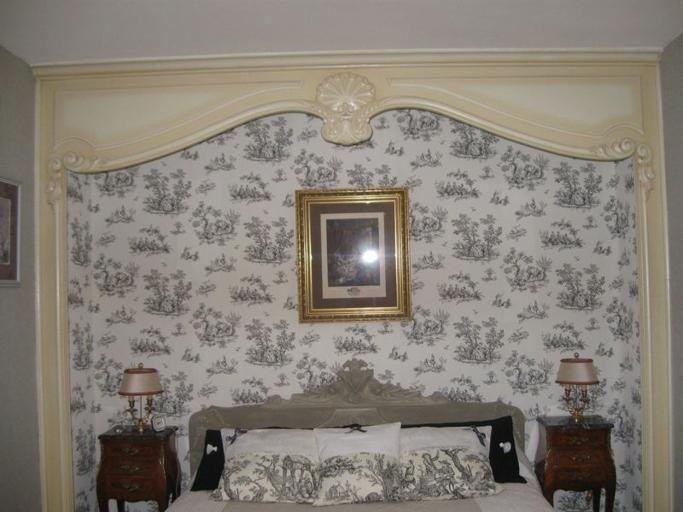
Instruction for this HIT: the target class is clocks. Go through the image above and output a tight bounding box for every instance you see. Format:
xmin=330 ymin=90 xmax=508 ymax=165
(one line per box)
xmin=151 ymin=414 xmax=167 ymax=432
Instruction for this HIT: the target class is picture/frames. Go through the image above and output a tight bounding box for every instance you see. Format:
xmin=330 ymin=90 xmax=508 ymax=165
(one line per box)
xmin=0 ymin=176 xmax=22 ymax=286
xmin=293 ymin=187 xmax=412 ymax=323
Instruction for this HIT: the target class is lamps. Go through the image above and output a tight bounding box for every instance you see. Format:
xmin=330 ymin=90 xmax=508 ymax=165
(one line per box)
xmin=118 ymin=362 xmax=164 ymax=433
xmin=555 ymin=352 xmax=601 ymax=424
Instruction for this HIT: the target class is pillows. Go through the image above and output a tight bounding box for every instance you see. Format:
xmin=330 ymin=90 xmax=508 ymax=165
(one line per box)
xmin=314 ymin=422 xmax=403 ymax=506
xmin=401 ymin=425 xmax=508 ymax=501
xmin=192 ymin=430 xmax=225 ymax=491
xmin=208 ymin=427 xmax=318 ymax=503
xmin=400 ymin=415 xmax=526 ymax=486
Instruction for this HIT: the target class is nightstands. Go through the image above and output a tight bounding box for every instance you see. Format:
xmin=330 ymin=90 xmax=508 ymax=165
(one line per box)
xmin=533 ymin=415 xmax=616 ymax=512
xmin=96 ymin=424 xmax=181 ymax=512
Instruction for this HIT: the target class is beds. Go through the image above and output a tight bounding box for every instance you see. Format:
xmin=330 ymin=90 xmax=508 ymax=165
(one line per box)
xmin=160 ymin=358 xmax=559 ymax=512
